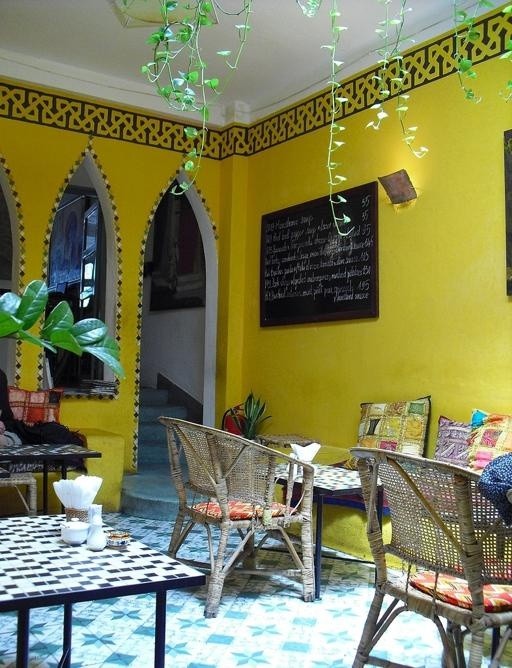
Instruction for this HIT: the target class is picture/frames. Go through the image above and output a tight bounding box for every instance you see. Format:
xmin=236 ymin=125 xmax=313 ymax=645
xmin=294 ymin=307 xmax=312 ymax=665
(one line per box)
xmin=46 ymin=195 xmax=89 ymax=292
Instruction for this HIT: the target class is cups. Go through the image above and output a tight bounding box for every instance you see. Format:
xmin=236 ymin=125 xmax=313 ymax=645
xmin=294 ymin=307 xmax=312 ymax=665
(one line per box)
xmin=60 ymin=507 xmax=92 ymax=546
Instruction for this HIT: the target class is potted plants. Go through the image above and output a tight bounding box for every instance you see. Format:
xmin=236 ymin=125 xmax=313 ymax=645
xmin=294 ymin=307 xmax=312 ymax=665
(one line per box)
xmin=227 ymin=387 xmax=271 ymax=442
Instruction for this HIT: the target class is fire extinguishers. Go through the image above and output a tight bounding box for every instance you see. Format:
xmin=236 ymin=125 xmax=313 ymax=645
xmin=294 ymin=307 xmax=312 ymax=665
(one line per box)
xmin=222 ymin=403 xmax=246 ymax=436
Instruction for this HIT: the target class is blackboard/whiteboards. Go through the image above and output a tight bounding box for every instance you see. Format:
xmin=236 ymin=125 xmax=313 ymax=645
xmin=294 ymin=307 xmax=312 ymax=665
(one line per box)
xmin=259 ymin=180 xmax=379 ymax=327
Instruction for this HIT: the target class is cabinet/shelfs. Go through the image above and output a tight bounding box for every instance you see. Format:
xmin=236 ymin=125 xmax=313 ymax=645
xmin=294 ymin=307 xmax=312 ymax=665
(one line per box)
xmin=80 ymin=200 xmax=108 ymax=379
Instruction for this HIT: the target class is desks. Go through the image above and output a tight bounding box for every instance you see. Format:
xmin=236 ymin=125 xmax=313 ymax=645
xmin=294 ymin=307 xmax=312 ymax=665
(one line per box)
xmin=257 ymin=462 xmax=383 ymax=601
xmin=0 ymin=442 xmax=102 ymax=513
xmin=0 ymin=515 xmax=206 ymax=668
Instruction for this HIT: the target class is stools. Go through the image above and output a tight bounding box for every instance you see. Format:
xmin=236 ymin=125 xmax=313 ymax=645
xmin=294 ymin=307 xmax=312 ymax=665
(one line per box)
xmin=0 ymin=471 xmax=36 ymax=518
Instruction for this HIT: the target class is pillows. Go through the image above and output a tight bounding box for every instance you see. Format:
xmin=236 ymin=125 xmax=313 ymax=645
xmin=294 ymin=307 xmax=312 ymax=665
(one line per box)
xmin=435 ymin=410 xmax=511 ymax=471
xmin=7 ymin=386 xmax=64 ymax=426
xmin=339 ymin=395 xmax=431 ymax=470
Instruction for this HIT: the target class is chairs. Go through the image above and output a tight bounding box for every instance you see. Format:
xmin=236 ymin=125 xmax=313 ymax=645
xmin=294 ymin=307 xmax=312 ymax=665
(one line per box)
xmin=156 ymin=414 xmax=314 ymax=618
xmin=348 ymin=445 xmax=512 ymax=668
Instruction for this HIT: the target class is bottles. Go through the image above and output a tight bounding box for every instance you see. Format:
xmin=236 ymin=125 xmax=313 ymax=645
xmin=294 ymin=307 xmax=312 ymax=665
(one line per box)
xmin=87 ymin=504 xmax=107 ymax=551
